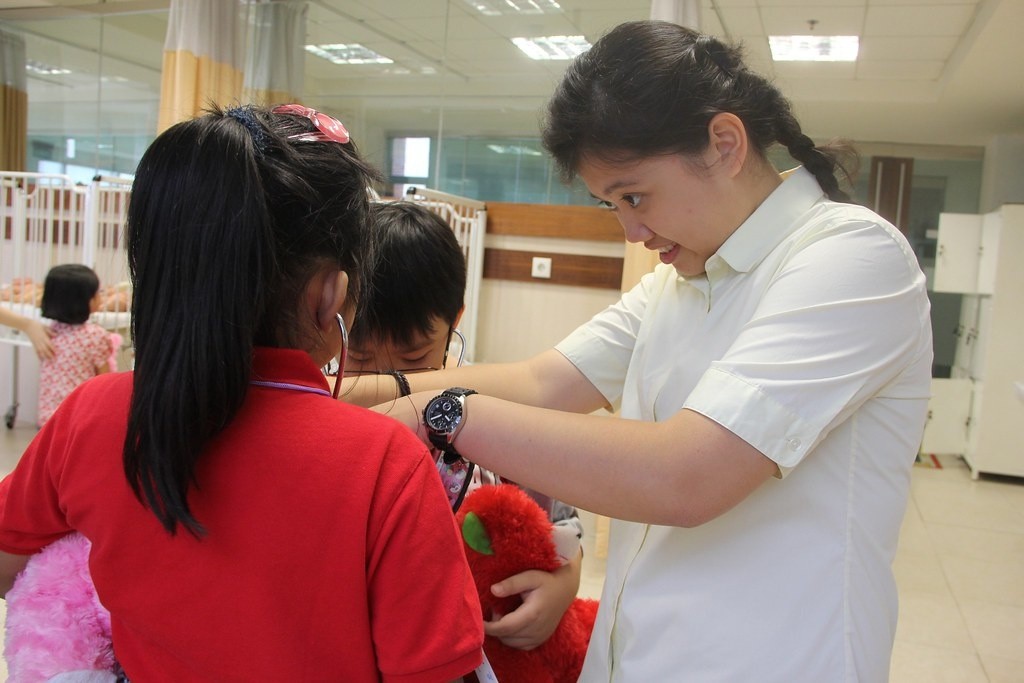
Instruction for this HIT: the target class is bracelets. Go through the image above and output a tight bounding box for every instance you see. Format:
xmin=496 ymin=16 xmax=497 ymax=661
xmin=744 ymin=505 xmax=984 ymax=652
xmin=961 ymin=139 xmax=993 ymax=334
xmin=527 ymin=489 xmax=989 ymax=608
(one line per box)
xmin=389 ymin=371 xmax=411 ymax=396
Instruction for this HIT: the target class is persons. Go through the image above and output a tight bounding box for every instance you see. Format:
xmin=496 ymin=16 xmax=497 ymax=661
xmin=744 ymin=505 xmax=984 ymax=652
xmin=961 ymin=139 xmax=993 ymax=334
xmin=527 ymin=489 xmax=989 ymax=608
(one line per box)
xmin=323 ymin=22 xmax=935 ymax=683
xmin=0 ymin=103 xmax=487 ymax=683
xmin=0 ymin=306 xmax=56 ymax=360
xmin=335 ymin=201 xmax=582 ymax=682
xmin=38 ymin=264 xmax=113 ymax=428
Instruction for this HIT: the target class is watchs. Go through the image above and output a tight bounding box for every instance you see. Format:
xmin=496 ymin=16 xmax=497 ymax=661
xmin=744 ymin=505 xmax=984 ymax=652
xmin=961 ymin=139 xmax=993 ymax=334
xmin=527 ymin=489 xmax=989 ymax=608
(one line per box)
xmin=422 ymin=387 xmax=477 ymax=464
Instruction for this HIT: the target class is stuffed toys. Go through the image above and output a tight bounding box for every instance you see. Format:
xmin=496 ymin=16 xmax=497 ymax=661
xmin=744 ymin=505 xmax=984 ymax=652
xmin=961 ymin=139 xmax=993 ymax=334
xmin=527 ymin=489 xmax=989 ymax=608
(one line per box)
xmin=4 ymin=532 xmax=127 ymax=683
xmin=108 ymin=334 xmax=122 ymax=372
xmin=457 ymin=484 xmax=600 ymax=683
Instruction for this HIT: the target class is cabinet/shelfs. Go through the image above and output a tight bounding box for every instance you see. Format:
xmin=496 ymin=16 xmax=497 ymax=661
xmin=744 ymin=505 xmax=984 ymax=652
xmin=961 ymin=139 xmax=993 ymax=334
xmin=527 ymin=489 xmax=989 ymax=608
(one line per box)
xmin=921 ymin=203 xmax=1024 ymax=482
